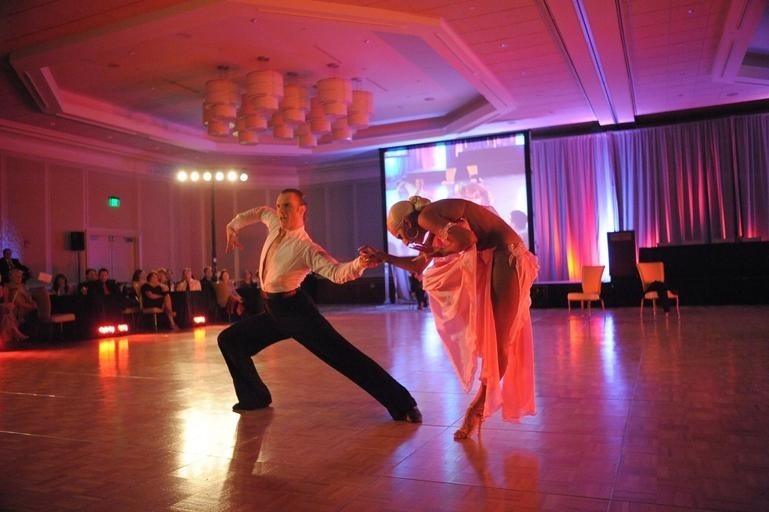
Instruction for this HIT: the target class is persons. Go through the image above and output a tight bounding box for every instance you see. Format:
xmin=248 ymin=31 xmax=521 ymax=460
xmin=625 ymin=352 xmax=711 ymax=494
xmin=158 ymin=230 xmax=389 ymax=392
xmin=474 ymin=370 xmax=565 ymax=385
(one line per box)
xmin=407 ymin=273 xmax=431 ymax=310
xmin=0 ymin=248 xmax=259 ymax=351
xmin=359 ymin=196 xmax=540 ymax=442
xmin=217 ymin=188 xmax=422 ymax=423
xmin=389 ymin=177 xmax=529 ymax=300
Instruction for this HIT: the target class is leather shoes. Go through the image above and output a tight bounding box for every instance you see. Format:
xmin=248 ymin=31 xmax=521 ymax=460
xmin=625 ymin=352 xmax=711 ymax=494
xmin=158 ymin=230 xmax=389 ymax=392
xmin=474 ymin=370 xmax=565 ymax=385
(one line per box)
xmin=404 ymin=405 xmax=422 ymax=423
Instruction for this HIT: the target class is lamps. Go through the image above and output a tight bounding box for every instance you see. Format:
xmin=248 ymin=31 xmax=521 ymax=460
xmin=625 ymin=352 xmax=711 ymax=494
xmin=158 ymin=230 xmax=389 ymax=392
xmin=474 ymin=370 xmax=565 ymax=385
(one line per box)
xmin=173 ymin=166 xmax=250 ymax=186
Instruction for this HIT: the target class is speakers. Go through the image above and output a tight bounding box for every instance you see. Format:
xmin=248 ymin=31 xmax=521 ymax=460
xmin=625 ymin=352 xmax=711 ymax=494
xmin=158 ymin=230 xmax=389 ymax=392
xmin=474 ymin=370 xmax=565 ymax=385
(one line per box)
xmin=71 ymin=232 xmax=84 ymax=251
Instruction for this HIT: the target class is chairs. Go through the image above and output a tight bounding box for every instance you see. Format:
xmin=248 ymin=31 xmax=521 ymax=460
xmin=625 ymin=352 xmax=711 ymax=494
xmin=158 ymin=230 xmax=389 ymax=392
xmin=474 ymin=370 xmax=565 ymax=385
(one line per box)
xmin=566 ymin=263 xmax=607 ymax=317
xmin=636 ymin=259 xmax=681 ymax=320
xmin=0 ymin=275 xmax=256 ymax=338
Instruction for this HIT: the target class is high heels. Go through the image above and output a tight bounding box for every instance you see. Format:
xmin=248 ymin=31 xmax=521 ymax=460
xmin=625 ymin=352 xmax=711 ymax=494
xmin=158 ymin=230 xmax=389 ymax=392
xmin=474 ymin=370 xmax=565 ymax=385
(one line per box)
xmin=453 ymin=403 xmax=484 ymax=440
